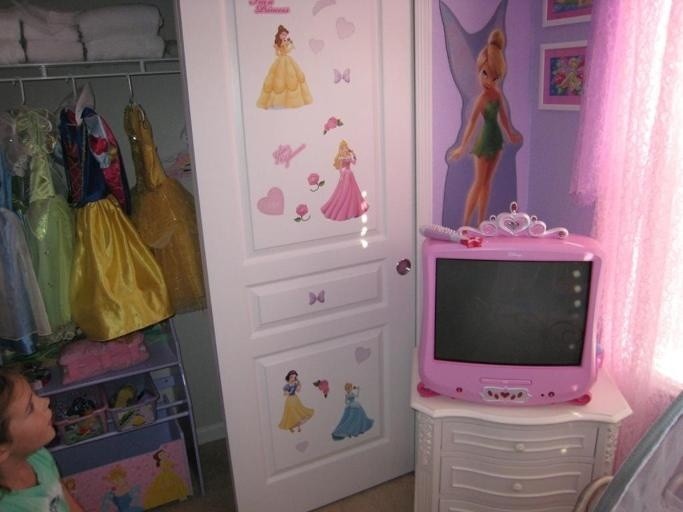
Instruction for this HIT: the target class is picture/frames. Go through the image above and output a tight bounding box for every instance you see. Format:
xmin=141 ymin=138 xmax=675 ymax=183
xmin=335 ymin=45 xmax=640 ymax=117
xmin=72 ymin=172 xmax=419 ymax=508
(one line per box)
xmin=541 ymin=0 xmax=593 ymax=27
xmin=539 ymin=39 xmax=591 ymax=111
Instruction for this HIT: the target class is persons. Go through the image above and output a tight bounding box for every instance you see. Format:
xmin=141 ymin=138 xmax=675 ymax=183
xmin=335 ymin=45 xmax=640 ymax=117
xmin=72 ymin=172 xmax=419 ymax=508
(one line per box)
xmin=146 ymin=450 xmax=189 ymax=509
xmin=331 ymin=383 xmax=374 ymax=440
xmin=276 ymin=370 xmax=315 ymax=432
xmin=447 ymin=27 xmax=521 ymax=226
xmin=0 ymin=361 xmax=86 ymax=512
xmin=559 ymin=57 xmax=579 ymax=96
xmin=320 ymin=141 xmax=371 ymax=222
xmin=61 ymin=477 xmax=82 ymax=511
xmin=257 ymin=23 xmax=312 ymax=111
xmin=101 ymin=468 xmax=145 ymax=509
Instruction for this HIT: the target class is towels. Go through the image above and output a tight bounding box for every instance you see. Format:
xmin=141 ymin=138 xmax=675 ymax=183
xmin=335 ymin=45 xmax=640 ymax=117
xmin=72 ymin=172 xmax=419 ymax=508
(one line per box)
xmin=0 ymin=4 xmax=166 ymax=65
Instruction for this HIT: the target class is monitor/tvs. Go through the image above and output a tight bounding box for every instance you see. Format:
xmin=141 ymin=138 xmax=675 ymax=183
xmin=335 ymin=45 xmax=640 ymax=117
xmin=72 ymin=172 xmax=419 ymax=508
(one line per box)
xmin=420 ymin=201 xmax=607 ymax=406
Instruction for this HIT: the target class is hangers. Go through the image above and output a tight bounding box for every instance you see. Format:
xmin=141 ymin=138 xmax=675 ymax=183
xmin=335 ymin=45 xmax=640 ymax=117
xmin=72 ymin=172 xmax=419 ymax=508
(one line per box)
xmin=10 ymin=76 xmax=145 ymax=133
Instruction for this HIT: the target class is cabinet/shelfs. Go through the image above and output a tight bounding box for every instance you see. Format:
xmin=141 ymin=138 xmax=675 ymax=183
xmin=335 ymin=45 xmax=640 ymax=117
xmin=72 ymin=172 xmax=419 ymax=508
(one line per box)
xmin=409 ymin=346 xmax=634 ymax=511
xmin=20 ymin=318 xmax=206 ymax=511
